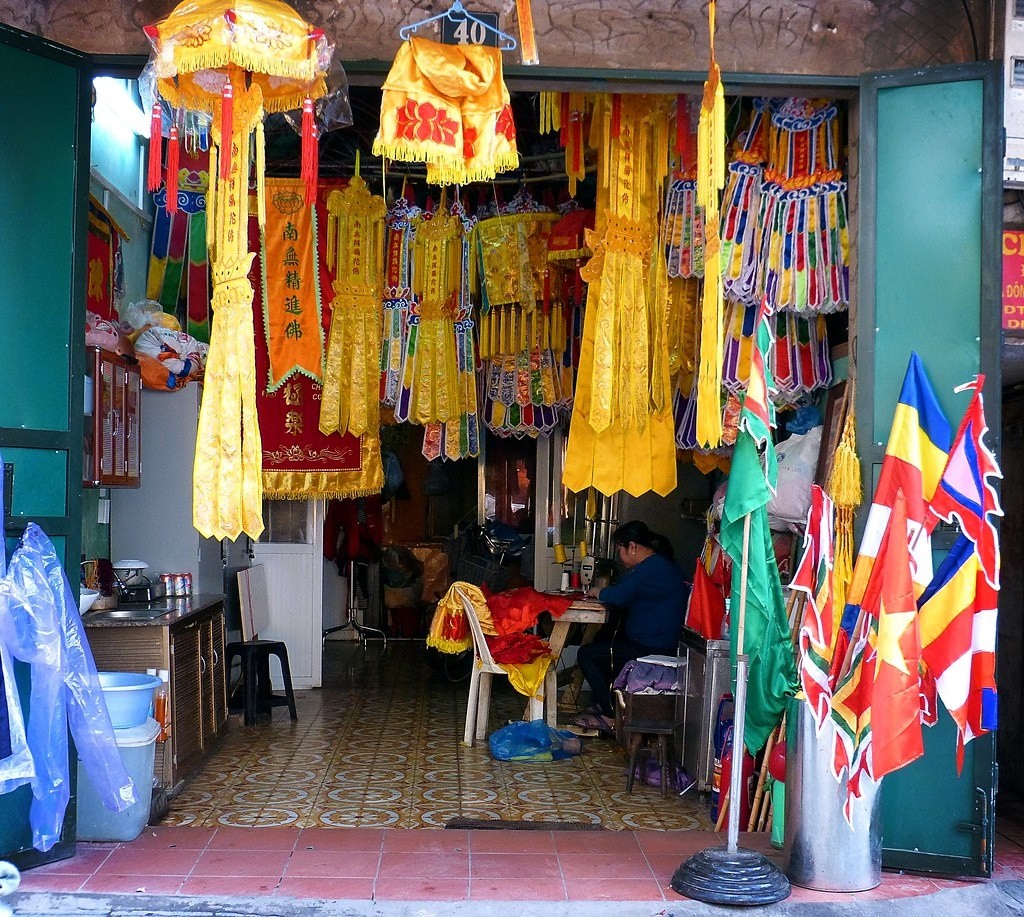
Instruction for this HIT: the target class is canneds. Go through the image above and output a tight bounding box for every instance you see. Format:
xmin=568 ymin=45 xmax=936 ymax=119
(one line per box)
xmin=160 ymin=573 xmax=193 ymax=596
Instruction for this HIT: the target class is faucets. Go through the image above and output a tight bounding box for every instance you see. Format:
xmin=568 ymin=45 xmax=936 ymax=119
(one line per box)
xmin=80 ymin=560 xmax=129 ymax=594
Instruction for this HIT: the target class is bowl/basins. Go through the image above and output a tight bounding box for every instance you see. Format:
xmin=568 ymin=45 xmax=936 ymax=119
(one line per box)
xmin=97 ymin=672 xmax=162 ymax=729
xmin=80 ymin=588 xmax=99 ymax=616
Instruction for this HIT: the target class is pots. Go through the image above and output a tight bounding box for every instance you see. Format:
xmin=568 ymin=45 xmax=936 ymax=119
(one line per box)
xmin=114 ymin=569 xmax=143 ymax=585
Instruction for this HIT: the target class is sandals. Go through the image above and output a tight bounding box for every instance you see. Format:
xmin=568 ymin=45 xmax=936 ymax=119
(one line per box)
xmin=575 ymin=714 xmax=618 ymax=736
xmin=580 ymin=702 xmax=604 ymax=714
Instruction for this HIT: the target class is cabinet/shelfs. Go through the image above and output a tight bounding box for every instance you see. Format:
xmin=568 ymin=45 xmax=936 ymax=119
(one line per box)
xmin=80 ymin=594 xmax=228 ymax=801
xmin=673 ymin=625 xmax=731 ymax=804
xmin=84 ymin=345 xmax=142 ymax=490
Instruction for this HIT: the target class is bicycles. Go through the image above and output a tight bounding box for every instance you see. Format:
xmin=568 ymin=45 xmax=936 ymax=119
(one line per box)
xmin=441 ymin=525 xmax=532 ymax=685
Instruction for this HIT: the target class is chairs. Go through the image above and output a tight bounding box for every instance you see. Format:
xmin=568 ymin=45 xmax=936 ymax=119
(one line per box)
xmin=453 ymin=585 xmax=544 ymax=746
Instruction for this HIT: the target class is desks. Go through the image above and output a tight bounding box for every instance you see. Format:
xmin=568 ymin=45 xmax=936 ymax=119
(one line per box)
xmin=505 ymin=588 xmax=609 ymax=739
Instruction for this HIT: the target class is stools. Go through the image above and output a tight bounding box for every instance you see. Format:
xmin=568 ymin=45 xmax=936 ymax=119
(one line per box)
xmin=622 ymin=721 xmax=684 ymax=799
xmin=225 ymin=640 xmax=298 ymax=728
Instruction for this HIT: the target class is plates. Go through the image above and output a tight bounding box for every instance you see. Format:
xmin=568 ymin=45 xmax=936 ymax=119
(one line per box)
xmin=113 ymin=559 xmax=148 ymax=568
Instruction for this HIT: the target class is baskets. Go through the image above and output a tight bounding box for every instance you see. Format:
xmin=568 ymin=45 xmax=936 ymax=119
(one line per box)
xmin=458 ymin=555 xmax=511 ymax=590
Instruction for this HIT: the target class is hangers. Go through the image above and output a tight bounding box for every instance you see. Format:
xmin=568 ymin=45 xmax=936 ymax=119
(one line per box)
xmin=399 ymin=0 xmax=517 ymax=51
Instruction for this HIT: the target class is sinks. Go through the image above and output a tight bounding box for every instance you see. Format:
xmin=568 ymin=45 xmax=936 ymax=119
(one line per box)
xmin=83 ymin=607 xmax=178 ymax=620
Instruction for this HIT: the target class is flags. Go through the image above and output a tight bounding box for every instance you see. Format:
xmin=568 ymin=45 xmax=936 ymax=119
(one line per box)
xmin=719 ymin=359 xmax=1005 ymax=827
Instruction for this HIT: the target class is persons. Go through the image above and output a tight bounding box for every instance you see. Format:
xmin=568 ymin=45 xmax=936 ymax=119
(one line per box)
xmin=572 ymin=520 xmax=690 ymax=731
